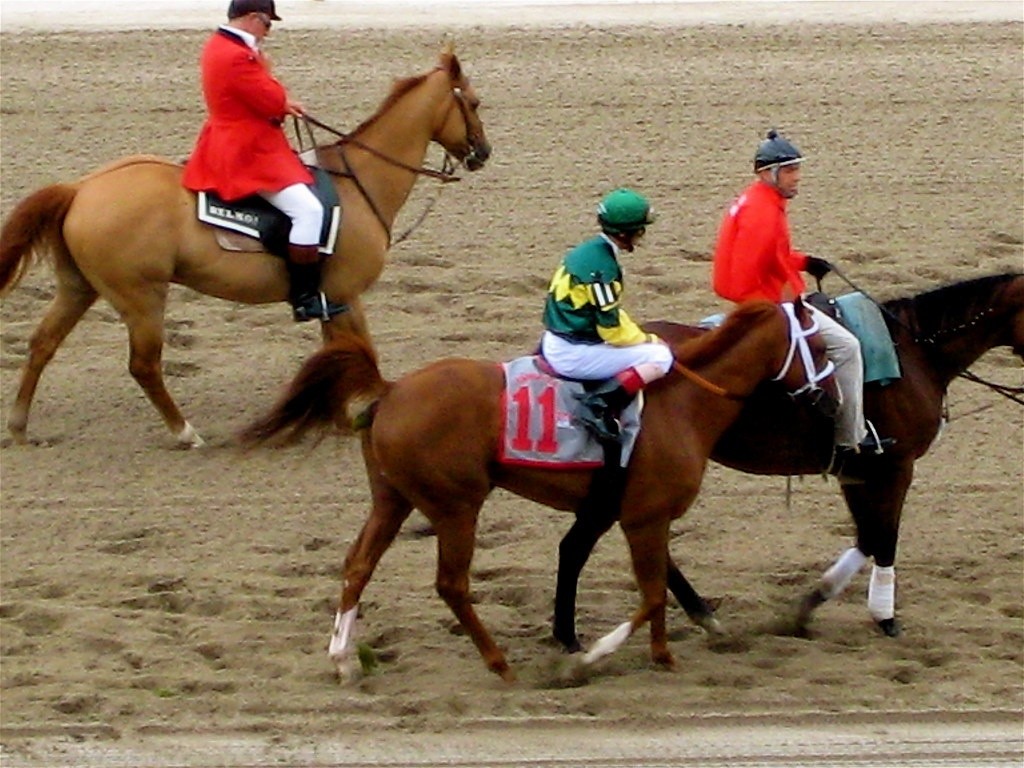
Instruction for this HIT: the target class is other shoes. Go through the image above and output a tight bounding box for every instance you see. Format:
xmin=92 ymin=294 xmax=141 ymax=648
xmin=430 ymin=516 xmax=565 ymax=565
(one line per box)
xmin=835 ymin=432 xmax=897 ymax=463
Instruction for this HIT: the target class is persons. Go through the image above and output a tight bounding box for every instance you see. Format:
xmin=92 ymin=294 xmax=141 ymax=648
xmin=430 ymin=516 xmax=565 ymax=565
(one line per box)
xmin=714 ymin=130 xmax=896 ymax=461
xmin=179 ymin=0 xmax=351 ymax=322
xmin=541 ymin=189 xmax=673 ymax=443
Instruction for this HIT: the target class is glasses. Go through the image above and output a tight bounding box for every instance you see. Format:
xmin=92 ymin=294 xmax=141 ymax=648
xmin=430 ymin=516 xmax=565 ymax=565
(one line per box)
xmin=633 ymin=227 xmax=646 ymax=236
xmin=258 ymin=14 xmax=273 ymax=31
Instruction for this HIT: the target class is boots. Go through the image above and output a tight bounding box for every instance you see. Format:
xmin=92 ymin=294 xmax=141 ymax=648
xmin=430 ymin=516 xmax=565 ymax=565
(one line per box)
xmin=287 ymin=243 xmax=350 ymax=322
xmin=572 ymin=367 xmax=648 ymax=444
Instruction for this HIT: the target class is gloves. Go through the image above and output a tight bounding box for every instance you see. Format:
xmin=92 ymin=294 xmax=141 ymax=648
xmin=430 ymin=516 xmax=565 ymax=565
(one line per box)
xmin=805 ymin=255 xmax=834 ymax=281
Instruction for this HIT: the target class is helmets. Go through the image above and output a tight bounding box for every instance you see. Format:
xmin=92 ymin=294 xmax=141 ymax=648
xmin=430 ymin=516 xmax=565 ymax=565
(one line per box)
xmin=597 ymin=187 xmax=660 ymax=234
xmin=228 ymin=0 xmax=282 ymax=21
xmin=753 ymin=128 xmax=806 ymax=173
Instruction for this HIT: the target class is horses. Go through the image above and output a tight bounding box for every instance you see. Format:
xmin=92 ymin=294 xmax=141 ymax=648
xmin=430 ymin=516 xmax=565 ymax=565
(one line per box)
xmin=1 ymin=45 xmax=492 ymax=450
xmin=197 ymin=294 xmax=843 ymax=687
xmin=407 ymin=273 xmax=1024 ymax=654
